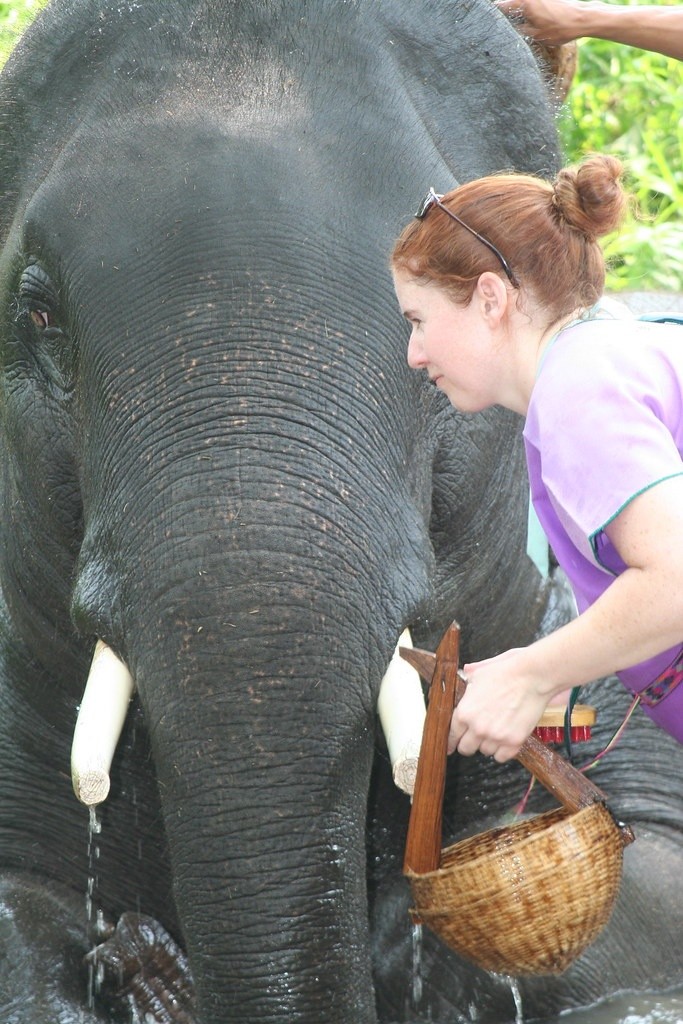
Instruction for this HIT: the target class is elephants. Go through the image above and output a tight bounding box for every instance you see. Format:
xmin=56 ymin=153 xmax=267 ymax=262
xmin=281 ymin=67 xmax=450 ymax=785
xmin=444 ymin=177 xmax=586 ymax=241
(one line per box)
xmin=0 ymin=0 xmax=682 ymax=1024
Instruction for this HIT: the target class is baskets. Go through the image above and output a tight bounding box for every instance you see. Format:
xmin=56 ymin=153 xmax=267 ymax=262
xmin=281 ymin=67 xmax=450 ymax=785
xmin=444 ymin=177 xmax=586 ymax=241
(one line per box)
xmin=397 ymin=623 xmax=633 ymax=978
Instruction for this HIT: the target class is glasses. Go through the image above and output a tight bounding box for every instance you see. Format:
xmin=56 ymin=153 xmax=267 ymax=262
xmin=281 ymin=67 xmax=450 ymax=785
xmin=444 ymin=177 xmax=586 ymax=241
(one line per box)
xmin=414 ymin=187 xmax=519 ymax=291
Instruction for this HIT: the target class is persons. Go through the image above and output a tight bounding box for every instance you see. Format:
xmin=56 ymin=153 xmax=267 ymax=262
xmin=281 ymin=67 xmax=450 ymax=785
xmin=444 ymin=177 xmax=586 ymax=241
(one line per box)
xmin=389 ymin=153 xmax=683 ymax=763
